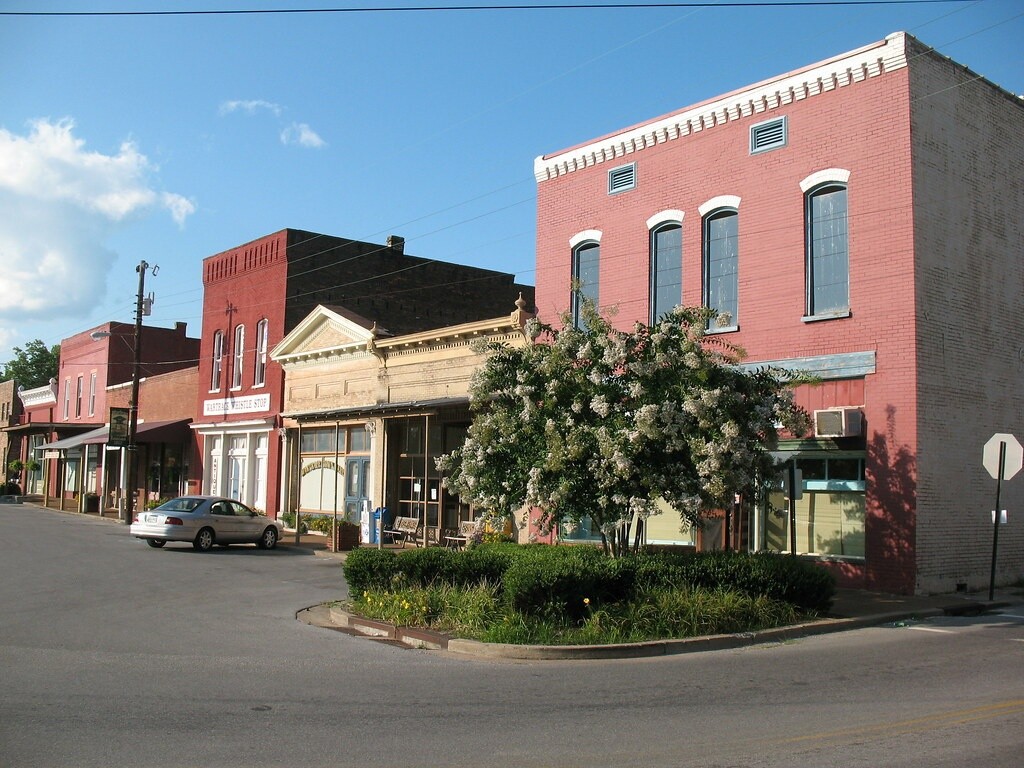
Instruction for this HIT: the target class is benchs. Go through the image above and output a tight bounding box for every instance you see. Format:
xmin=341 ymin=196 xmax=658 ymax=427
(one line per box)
xmin=382 ymin=516 xmax=420 ymax=548
xmin=443 ymin=521 xmax=476 ymax=551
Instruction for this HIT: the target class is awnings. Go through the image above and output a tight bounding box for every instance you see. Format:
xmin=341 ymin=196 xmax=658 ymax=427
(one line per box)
xmin=0 ymin=417 xmax=193 ymax=451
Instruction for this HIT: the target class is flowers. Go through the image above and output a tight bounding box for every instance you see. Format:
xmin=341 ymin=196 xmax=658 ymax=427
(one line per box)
xmin=75 ymin=491 xmax=100 ymax=504
xmin=328 ymin=520 xmax=355 ymax=539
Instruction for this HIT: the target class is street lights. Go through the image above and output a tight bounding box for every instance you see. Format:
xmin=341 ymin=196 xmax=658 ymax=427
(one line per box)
xmin=89 ymin=259 xmax=161 ymax=527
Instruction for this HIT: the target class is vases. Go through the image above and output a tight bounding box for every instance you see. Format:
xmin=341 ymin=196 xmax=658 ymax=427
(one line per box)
xmin=87 ymin=499 xmax=98 ymax=511
xmin=326 ymin=527 xmax=360 ymax=551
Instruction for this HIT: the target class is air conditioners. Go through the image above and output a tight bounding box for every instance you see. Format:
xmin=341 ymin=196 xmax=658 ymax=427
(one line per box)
xmin=814 ymin=409 xmax=862 ymax=437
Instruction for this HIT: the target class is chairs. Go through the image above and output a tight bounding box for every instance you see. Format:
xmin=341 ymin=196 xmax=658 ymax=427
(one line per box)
xmin=213 ymin=506 xmax=222 ymax=513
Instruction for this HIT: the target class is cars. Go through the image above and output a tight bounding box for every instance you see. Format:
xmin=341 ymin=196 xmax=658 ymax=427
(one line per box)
xmin=130 ymin=495 xmax=285 ymax=554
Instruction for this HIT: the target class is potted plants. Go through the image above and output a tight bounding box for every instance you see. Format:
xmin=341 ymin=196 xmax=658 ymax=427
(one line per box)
xmin=278 ymin=512 xmax=337 ymax=536
xmin=144 ymin=500 xmax=157 ymax=511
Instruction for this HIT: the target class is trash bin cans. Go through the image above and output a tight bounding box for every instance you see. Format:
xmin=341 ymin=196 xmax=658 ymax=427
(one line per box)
xmin=374 ymin=507 xmax=387 ymax=543
